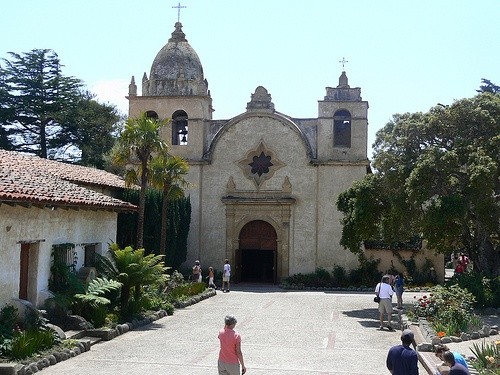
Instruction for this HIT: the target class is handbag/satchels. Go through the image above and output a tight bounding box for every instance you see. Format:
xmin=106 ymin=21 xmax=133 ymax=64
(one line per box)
xmin=374 ymin=297 xmax=380 ymax=302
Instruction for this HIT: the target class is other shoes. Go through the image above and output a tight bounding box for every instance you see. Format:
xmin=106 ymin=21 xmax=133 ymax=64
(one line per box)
xmin=387 ymin=325 xmax=393 ymax=330
xmin=220 ymin=289 xmax=224 ymax=291
xmin=226 ymin=290 xmax=229 ymax=291
xmin=380 ymin=326 xmax=383 ymax=329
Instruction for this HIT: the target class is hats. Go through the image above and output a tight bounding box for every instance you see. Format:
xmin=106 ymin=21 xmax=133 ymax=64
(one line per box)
xmin=402 ymin=329 xmax=414 ymax=342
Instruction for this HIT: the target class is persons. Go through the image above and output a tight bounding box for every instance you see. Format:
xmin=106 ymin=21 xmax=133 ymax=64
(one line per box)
xmin=218 ymin=314 xmax=247 ymax=375
xmin=205 ymin=267 xmax=217 ymax=290
xmin=387 ymin=329 xmax=419 ymax=375
xmin=375 ymin=276 xmax=393 ymax=330
xmin=432 ymin=345 xmax=471 ymax=375
xmin=192 ymin=260 xmax=202 ymax=282
xmin=383 ymin=269 xmax=408 ymax=310
xmin=454 ymin=252 xmax=470 ymax=274
xmin=220 ymin=259 xmax=231 ymax=292
